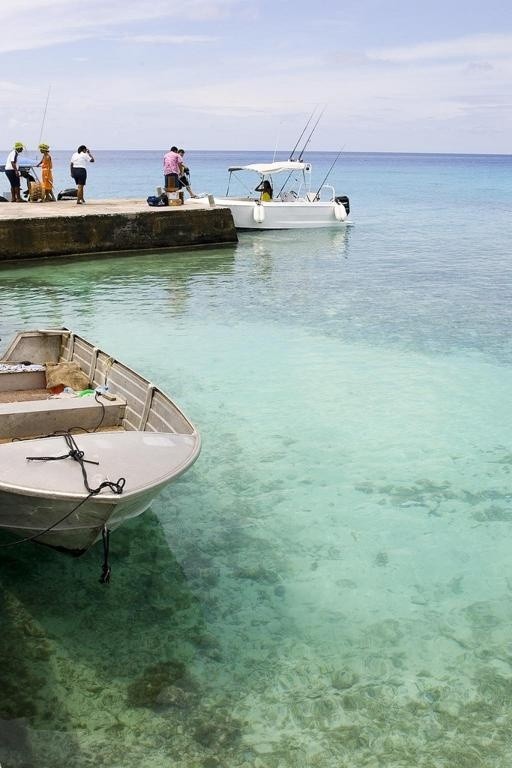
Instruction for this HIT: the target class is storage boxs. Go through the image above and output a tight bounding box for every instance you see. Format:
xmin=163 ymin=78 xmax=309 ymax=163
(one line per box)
xmin=166 ymin=191 xmax=184 ymax=206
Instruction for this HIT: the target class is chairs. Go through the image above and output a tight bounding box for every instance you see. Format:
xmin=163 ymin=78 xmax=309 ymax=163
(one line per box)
xmin=279 ymin=192 xmax=296 ymax=202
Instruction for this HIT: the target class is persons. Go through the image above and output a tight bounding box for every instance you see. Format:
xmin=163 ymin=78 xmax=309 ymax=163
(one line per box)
xmin=255 ymin=180 xmax=273 ymax=200
xmin=163 ymin=146 xmax=197 ymax=198
xmin=5 ymin=141 xmax=27 ymax=202
xmin=36 ymin=143 xmax=56 ymax=202
xmin=70 ymin=145 xmax=95 ymax=204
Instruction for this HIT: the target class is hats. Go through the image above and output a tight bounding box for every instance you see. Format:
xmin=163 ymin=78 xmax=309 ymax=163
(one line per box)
xmin=15 ymin=142 xmax=49 ymax=152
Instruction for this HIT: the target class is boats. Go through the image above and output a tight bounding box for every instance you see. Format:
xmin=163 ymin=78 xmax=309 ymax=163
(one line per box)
xmin=0 ymin=157 xmax=78 ymax=202
xmin=0 ymin=326 xmax=202 ymax=555
xmin=184 ymin=161 xmax=355 ymax=229
